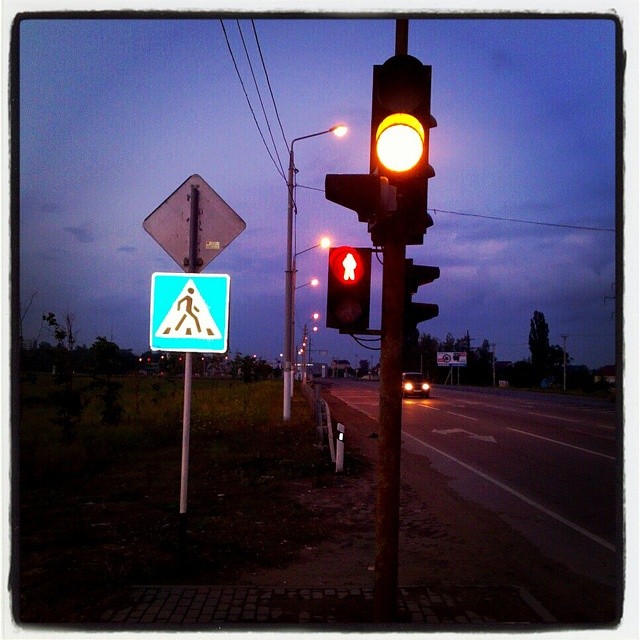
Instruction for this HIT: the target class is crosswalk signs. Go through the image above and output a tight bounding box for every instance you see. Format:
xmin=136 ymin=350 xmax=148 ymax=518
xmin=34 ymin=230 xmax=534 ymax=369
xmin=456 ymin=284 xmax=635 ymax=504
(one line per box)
xmin=150 ymin=273 xmax=230 ymax=354
xmin=143 ymin=174 xmax=247 ymax=273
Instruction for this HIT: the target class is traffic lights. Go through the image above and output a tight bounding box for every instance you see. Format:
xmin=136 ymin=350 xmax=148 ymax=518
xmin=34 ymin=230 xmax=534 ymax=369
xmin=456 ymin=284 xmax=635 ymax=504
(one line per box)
xmin=405 ymin=257 xmax=439 ymax=372
xmin=325 ymin=55 xmax=438 ymax=245
xmin=326 ymin=248 xmax=372 ymax=335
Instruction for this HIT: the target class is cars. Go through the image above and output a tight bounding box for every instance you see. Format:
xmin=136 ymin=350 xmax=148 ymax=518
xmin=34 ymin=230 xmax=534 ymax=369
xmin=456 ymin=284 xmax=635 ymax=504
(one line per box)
xmin=402 ymin=373 xmax=430 ymax=398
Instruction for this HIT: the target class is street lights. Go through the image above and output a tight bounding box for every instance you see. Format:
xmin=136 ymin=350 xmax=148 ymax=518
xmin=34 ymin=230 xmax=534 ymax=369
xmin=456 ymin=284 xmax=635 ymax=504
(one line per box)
xmin=295 ymin=279 xmax=318 ymax=289
xmin=283 ymin=123 xmax=349 ymax=420
xmin=302 ymin=327 xmax=318 ymax=384
xmin=303 ymin=314 xmax=319 ymax=384
xmin=291 ymin=238 xmax=329 ymax=396
